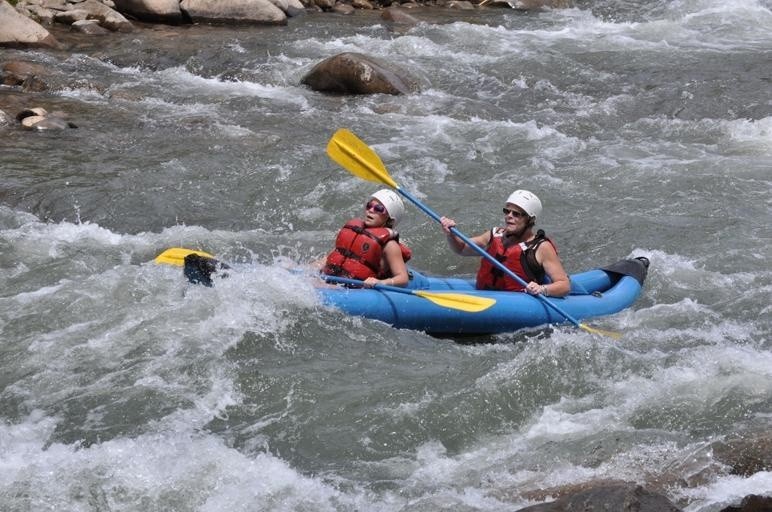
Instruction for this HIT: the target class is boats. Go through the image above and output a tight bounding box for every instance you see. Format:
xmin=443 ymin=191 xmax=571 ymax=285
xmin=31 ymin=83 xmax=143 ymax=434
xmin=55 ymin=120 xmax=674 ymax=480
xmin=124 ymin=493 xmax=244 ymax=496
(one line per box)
xmin=152 ymin=247 xmax=653 ymax=335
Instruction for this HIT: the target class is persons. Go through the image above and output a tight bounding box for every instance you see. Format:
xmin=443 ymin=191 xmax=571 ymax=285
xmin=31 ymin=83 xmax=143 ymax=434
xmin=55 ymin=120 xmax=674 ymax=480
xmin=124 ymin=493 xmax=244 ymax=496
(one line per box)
xmin=318 ymin=188 xmax=412 ymax=289
xmin=439 ymin=189 xmax=571 ymax=298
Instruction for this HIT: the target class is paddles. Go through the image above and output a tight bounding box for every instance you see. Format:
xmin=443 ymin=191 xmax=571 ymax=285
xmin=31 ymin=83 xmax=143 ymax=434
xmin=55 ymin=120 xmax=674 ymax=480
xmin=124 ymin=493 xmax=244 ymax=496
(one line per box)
xmin=327 ymin=129 xmax=621 ymax=342
xmin=154 ymin=248 xmax=496 ymax=313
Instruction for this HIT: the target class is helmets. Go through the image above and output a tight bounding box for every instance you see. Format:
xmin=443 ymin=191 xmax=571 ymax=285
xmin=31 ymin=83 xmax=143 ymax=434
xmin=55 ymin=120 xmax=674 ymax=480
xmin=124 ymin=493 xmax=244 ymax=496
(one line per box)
xmin=505 ymin=190 xmax=542 ymax=225
xmin=369 ymin=188 xmax=405 ymax=230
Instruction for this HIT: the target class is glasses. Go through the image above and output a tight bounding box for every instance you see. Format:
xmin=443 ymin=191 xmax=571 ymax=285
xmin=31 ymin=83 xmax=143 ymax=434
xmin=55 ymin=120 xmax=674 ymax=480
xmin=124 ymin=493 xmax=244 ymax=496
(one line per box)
xmin=367 ymin=202 xmax=388 ymax=218
xmin=503 ymin=207 xmax=528 ymax=217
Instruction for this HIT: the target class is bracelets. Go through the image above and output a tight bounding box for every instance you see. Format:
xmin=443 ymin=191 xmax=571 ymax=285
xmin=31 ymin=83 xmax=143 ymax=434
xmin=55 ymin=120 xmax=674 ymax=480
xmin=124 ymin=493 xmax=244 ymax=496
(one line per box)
xmin=386 ymin=278 xmax=394 ymax=286
xmin=541 ymin=284 xmax=548 ymax=297
